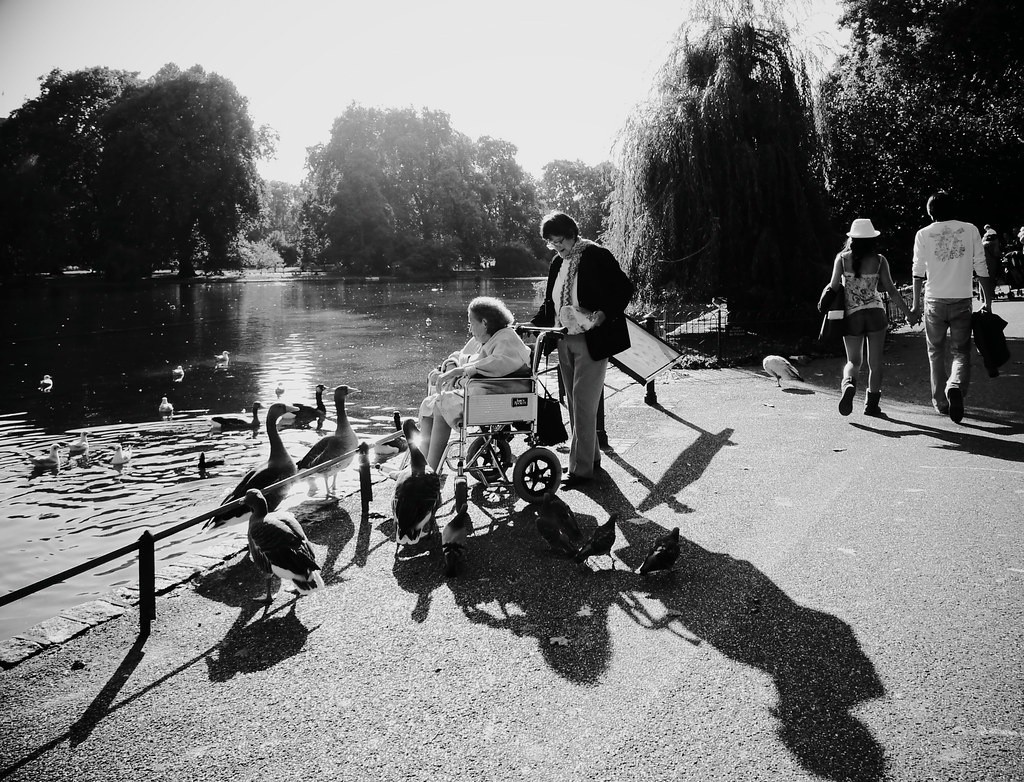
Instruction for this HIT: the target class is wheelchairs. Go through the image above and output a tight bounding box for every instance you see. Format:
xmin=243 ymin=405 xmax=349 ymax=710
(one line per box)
xmin=374 ymin=326 xmax=569 ymax=504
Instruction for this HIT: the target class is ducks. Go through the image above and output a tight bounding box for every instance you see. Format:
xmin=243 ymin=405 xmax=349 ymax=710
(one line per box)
xmin=25 ymin=350 xmax=473 ymax=604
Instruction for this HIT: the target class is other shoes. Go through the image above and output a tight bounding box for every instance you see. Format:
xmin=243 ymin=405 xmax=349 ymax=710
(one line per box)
xmin=560 ymin=472 xmax=592 ymax=484
xmin=944 ymin=384 xmax=965 ymax=424
xmin=389 ymin=464 xmax=436 ymax=481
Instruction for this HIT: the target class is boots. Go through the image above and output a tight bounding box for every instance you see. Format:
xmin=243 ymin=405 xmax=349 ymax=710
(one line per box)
xmin=838 ymin=375 xmax=857 ymax=416
xmin=864 ymin=388 xmax=882 ymax=414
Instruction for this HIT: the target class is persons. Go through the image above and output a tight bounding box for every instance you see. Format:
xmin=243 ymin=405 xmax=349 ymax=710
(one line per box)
xmin=830 ymin=218 xmax=918 ymax=416
xmin=906 ymin=192 xmax=992 ymax=424
xmin=389 ymin=298 xmax=531 ymax=481
xmin=514 ymin=212 xmax=634 ymax=484
xmin=972 ymin=224 xmax=1024 ymax=314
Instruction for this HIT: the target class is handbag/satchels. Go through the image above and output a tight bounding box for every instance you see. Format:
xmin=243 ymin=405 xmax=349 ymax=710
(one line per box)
xmin=817 ymin=254 xmax=849 ymax=341
xmin=530 ymin=379 xmax=569 ymax=446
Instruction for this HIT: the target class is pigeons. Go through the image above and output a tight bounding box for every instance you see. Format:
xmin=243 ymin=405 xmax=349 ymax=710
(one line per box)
xmin=535 ymin=490 xmax=680 ymax=576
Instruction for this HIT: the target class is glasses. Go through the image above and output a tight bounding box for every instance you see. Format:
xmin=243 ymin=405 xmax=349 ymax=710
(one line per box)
xmin=546 ymin=236 xmax=565 ymax=250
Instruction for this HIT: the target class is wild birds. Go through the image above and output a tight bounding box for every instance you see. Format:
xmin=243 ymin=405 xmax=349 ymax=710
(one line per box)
xmin=762 ymin=354 xmax=805 ymax=388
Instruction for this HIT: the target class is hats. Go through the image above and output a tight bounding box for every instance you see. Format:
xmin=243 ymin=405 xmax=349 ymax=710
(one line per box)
xmin=845 ymin=218 xmax=881 ymax=239
